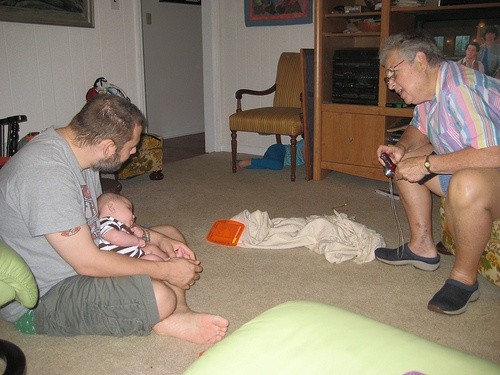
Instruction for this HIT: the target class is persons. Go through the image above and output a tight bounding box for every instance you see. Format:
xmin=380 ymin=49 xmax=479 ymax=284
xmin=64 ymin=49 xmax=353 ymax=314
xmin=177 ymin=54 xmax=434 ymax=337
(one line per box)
xmin=0 ymin=93 xmax=228 ymax=344
xmin=97 ymin=193 xmax=169 ymax=261
xmin=374 ymin=35 xmax=500 ymax=315
xmin=456 ymin=25 xmax=500 ymax=80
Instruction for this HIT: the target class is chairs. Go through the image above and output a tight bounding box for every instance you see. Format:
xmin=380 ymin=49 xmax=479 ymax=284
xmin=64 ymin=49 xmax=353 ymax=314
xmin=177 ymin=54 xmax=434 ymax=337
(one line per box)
xmin=0 ymin=113 xmax=28 ymax=169
xmin=0 ymin=234 xmax=40 ymax=375
xmin=229 ymin=51 xmax=304 ymax=182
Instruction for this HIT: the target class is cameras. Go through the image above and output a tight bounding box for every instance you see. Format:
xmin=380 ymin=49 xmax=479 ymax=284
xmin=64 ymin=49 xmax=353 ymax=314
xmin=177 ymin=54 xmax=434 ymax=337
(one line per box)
xmin=380 ymin=152 xmax=398 ymax=177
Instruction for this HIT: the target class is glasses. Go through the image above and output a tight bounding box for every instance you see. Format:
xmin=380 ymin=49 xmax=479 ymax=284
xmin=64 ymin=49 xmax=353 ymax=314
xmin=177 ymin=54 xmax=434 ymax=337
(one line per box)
xmin=384 ymin=59 xmax=406 ymax=79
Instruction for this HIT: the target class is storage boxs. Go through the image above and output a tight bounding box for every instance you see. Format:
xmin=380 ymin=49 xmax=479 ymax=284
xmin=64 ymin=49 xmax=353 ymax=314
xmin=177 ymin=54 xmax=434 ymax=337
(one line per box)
xmin=361 ymin=22 xmax=381 ymax=32
xmin=116 ymin=136 xmax=165 ymax=180
xmin=344 ymin=6 xmax=369 ymax=13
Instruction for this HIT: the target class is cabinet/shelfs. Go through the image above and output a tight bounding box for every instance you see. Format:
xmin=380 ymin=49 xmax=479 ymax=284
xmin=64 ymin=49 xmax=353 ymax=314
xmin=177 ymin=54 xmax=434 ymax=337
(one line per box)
xmin=313 ymin=0 xmax=500 ymax=181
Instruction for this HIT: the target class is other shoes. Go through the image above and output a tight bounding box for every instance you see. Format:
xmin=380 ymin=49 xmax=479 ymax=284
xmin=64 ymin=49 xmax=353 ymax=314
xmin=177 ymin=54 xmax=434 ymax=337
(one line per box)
xmin=375 ymin=241 xmax=441 ymax=271
xmin=428 ymin=278 xmax=480 ymax=315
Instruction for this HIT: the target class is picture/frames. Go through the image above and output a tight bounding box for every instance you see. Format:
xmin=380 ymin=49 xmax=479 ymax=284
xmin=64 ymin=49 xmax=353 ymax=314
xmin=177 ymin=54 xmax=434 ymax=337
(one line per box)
xmin=244 ymin=0 xmax=313 ymax=26
xmin=0 ymin=0 xmax=95 ymax=28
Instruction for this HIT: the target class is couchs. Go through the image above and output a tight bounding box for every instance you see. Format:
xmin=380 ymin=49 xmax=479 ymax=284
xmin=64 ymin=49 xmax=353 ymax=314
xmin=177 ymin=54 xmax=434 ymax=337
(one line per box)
xmin=437 ymin=194 xmax=500 ymax=288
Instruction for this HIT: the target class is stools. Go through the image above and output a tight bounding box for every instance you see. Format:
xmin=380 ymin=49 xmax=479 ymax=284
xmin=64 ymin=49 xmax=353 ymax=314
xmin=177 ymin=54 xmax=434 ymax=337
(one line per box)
xmin=181 ymin=298 xmax=500 ymax=375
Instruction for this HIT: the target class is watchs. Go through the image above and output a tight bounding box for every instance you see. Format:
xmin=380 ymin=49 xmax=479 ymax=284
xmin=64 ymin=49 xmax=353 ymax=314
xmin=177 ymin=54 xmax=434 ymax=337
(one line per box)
xmin=424 ymin=156 xmax=433 ymax=174
